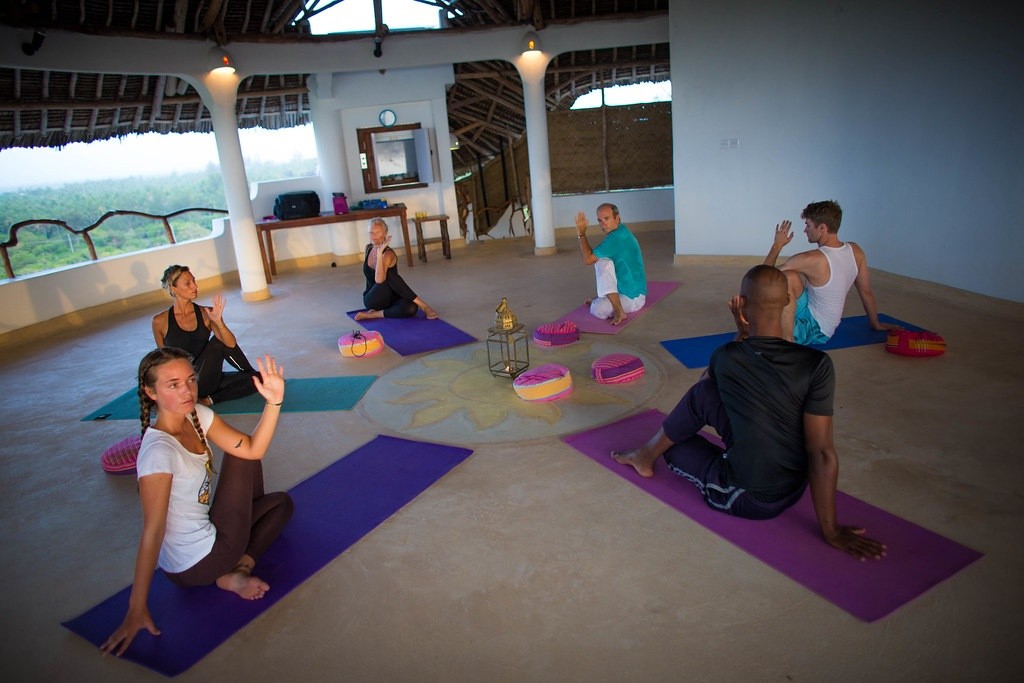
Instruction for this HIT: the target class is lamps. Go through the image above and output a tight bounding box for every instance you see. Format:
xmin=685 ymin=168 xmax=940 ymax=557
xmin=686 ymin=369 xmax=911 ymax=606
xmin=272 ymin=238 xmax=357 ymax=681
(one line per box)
xmin=450 ymin=132 xmax=460 ymax=150
xmin=207 ymin=46 xmax=235 ymax=74
xmin=521 ymin=31 xmax=542 ymax=58
xmin=486 ymin=296 xmax=529 ymax=379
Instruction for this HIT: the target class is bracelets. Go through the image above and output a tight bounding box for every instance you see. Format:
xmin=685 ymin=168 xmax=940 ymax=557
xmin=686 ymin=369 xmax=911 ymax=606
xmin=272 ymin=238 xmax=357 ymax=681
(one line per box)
xmin=577 ymin=235 xmax=584 ymax=239
xmin=266 ymin=402 xmax=283 ymax=406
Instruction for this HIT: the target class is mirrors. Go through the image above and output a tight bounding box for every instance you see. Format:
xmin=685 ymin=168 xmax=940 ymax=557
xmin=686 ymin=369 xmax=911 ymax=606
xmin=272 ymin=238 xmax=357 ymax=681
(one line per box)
xmin=379 ymin=110 xmax=396 ymax=127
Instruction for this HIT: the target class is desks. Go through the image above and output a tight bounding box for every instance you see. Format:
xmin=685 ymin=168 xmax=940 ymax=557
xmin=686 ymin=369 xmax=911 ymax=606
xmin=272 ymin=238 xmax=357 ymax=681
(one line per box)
xmin=412 ymin=214 xmax=452 ymax=262
xmin=255 ymin=203 xmax=414 ymax=284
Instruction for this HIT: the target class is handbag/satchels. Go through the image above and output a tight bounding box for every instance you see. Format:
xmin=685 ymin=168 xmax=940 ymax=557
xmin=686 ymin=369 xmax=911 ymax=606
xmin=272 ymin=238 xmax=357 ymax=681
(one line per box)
xmin=273 ymin=191 xmax=320 ymax=220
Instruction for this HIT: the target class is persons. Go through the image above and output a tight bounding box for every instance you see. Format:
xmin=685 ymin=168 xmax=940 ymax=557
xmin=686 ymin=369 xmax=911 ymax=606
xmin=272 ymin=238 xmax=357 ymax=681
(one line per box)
xmin=610 ymin=265 xmax=888 ymax=562
xmin=100 ymin=346 xmax=295 ymax=658
xmin=576 ymin=204 xmax=648 ymax=325
xmin=354 ymin=217 xmax=438 ymax=320
xmin=151 ymin=265 xmax=263 ymax=407
xmin=764 ymin=201 xmax=903 ymax=345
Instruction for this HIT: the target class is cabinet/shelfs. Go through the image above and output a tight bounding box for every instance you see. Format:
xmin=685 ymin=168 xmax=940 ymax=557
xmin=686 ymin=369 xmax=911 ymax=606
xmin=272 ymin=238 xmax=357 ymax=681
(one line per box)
xmin=356 ymin=121 xmax=435 ymax=194
